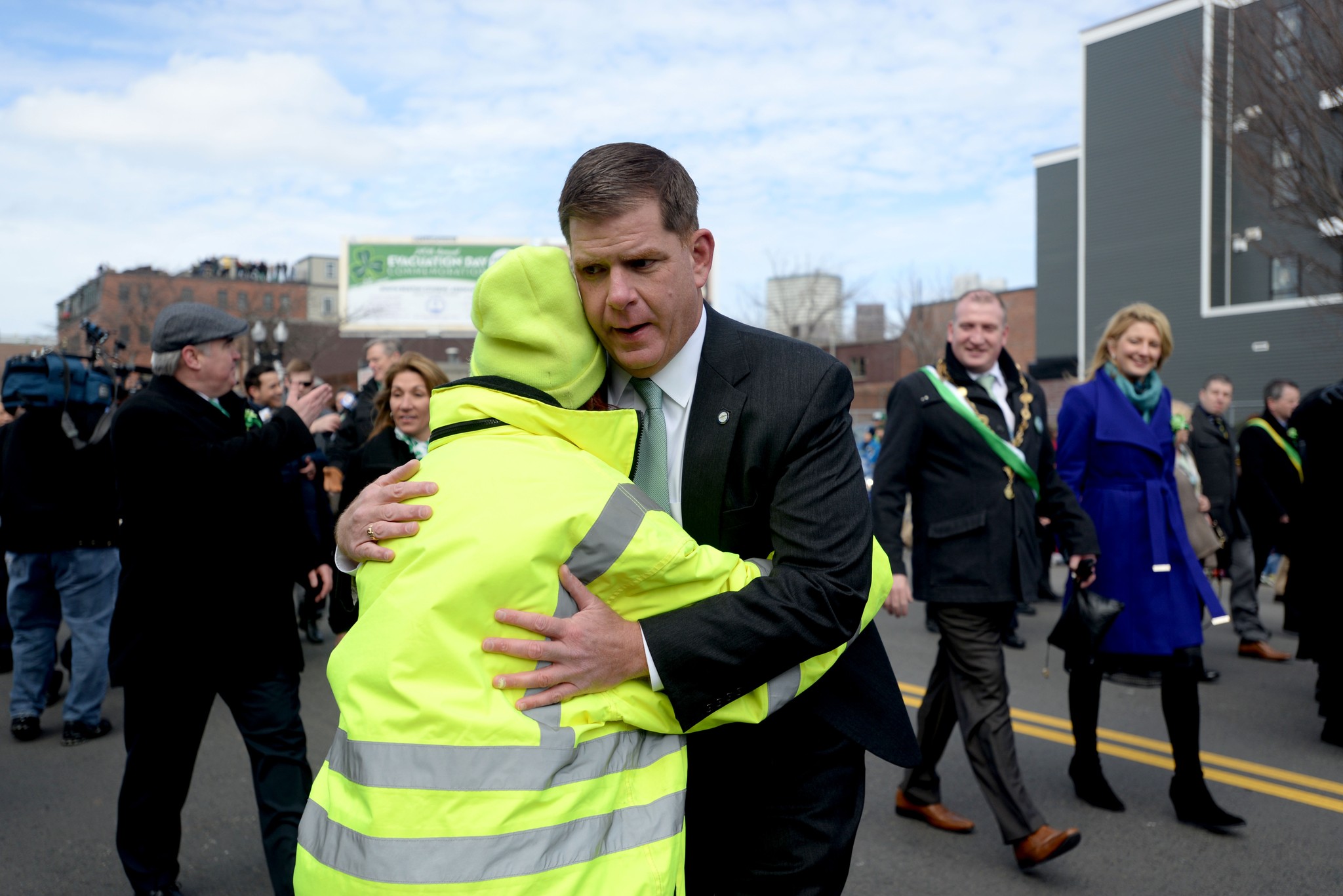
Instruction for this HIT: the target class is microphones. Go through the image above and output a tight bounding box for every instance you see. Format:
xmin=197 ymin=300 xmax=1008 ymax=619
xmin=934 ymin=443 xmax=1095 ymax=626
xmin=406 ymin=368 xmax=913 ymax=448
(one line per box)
xmin=131 ymin=365 xmax=153 ymax=375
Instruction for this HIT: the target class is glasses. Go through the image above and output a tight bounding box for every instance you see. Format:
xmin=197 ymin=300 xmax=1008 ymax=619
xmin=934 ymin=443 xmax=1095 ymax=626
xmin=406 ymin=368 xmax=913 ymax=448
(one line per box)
xmin=299 ymin=382 xmax=312 ymax=386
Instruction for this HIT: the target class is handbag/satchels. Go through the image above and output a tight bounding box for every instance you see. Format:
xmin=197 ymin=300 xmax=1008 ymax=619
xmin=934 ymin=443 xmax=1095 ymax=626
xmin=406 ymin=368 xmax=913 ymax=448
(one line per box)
xmin=1047 ymin=587 xmax=1124 ymax=658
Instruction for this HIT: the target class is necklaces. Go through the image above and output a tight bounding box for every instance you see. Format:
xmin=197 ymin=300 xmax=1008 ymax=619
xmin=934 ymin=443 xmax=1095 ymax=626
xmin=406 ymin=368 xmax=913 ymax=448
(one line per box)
xmin=935 ymin=360 xmax=1034 ymax=500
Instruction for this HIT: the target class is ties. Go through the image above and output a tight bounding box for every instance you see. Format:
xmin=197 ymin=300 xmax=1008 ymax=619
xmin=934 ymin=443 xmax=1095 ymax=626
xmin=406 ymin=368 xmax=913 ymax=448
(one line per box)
xmin=976 ymin=374 xmax=994 ymax=402
xmin=633 ymin=378 xmax=671 ymax=520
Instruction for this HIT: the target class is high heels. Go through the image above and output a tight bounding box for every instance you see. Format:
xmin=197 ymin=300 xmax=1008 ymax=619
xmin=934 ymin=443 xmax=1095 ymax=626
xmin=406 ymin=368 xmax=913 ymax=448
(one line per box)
xmin=1068 ymin=756 xmax=1122 ymax=809
xmin=1169 ymin=778 xmax=1246 ymax=829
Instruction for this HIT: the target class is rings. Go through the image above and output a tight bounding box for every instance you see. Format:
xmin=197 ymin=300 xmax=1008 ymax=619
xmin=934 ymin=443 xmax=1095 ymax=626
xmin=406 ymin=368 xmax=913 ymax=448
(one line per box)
xmin=367 ymin=524 xmax=380 ymax=541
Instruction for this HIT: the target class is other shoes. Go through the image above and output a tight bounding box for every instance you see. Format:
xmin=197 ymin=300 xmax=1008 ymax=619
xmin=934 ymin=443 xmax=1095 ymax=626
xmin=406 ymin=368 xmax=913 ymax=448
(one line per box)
xmin=300 ymin=615 xmax=318 ymax=642
xmin=63 ymin=717 xmax=111 ymax=745
xmin=10 ymin=717 xmax=41 ymax=740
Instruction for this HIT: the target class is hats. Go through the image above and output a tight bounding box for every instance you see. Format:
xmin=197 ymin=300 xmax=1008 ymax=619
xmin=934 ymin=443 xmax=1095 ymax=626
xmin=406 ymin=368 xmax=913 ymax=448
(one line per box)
xmin=151 ymin=302 xmax=250 ymax=352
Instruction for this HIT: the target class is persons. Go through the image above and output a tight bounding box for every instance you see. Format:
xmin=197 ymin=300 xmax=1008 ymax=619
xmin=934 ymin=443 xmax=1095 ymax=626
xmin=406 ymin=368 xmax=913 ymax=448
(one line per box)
xmin=856 ymin=411 xmax=888 ymax=504
xmin=1169 ymin=373 xmax=1294 ymax=681
xmin=105 ymin=303 xmax=333 ymax=896
xmin=0 ymin=334 xmax=411 ymax=744
xmin=329 ymin=352 xmax=451 ymax=645
xmin=334 ymin=142 xmax=923 ymax=896
xmin=1056 ymin=303 xmax=1247 ymax=829
xmin=1287 ymin=381 xmax=1343 ymax=748
xmin=293 ymin=246 xmax=893 ymax=896
xmin=872 ymin=289 xmax=1101 ymax=872
xmin=1236 ymin=380 xmax=1298 ymax=635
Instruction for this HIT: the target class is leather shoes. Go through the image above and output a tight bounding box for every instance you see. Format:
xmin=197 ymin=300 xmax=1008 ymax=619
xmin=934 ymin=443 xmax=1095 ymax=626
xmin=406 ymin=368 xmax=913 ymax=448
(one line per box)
xmin=893 ymin=789 xmax=973 ymax=832
xmin=1014 ymin=823 xmax=1080 ymax=869
xmin=1237 ymin=639 xmax=1291 ymax=661
xmin=1205 ymin=669 xmax=1221 ymax=684
xmin=1003 ymin=634 xmax=1026 ymax=649
